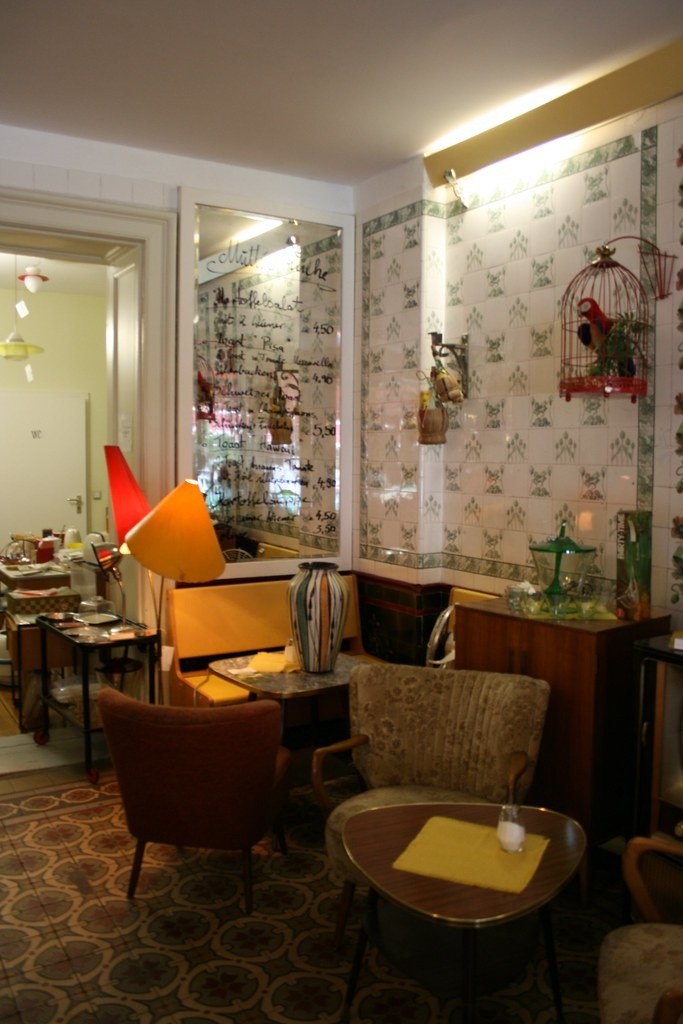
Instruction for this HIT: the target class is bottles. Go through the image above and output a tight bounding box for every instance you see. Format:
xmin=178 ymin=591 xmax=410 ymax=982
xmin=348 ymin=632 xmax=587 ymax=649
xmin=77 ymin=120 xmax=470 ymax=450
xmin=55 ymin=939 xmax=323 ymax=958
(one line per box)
xmin=38 ymin=529 xmax=55 ymax=562
xmin=496 ymin=805 xmax=525 ymax=852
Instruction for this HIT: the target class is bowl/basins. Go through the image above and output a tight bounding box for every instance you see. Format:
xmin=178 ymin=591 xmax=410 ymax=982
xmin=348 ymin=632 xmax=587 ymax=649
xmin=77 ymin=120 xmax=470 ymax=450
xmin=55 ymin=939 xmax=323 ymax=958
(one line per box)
xmin=46 ymin=611 xmax=72 ymax=622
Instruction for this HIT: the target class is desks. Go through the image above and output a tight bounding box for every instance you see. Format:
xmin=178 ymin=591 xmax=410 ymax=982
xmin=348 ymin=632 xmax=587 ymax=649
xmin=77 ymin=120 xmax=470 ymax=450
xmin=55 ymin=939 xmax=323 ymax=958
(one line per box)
xmin=0 ymin=556 xmax=81 ymax=734
xmin=209 ymin=650 xmax=363 ymax=703
xmin=340 ymin=804 xmax=587 ymax=1024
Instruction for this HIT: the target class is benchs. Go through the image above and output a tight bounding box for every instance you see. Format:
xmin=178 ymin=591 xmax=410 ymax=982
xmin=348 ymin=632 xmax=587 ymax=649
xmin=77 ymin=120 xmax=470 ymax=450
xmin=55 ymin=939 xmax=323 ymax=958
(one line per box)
xmin=167 ymin=575 xmax=385 ymax=708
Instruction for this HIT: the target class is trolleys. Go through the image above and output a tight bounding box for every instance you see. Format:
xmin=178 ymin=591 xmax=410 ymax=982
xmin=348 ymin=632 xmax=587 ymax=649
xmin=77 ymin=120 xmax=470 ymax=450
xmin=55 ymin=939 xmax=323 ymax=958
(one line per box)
xmin=32 ymin=612 xmax=158 ymax=784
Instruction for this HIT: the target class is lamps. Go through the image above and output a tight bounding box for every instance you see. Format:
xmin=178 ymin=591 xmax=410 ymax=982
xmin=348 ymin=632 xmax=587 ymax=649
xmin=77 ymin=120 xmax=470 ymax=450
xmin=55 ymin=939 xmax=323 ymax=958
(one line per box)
xmin=103 ymin=444 xmax=226 ymax=706
xmin=91 ymin=542 xmax=135 ymax=635
xmin=17 ymin=267 xmax=49 ymax=294
xmin=0 ymin=254 xmax=45 ymax=361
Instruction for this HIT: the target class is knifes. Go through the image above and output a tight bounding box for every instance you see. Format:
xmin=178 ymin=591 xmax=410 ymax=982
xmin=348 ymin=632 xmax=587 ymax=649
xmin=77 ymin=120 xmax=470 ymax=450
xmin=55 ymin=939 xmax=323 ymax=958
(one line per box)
xmin=65 ymin=632 xmax=98 ymax=636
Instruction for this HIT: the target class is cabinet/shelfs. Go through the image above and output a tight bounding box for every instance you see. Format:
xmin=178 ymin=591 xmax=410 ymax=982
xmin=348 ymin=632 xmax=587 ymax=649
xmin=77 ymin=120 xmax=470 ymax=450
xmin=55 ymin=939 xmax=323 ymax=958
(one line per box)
xmin=33 ymin=613 xmax=158 ymax=783
xmin=456 ymin=597 xmax=671 ymax=884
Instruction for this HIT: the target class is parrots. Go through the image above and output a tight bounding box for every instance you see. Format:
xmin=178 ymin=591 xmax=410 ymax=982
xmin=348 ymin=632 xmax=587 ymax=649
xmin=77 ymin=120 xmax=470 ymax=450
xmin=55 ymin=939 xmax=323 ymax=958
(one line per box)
xmin=576 ymin=298 xmax=630 ymax=376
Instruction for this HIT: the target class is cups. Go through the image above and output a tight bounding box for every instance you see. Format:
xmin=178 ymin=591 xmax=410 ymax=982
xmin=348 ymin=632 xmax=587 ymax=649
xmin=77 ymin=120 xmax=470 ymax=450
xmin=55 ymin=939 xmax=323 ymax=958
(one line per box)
xmin=504 ymin=583 xmax=598 ymax=622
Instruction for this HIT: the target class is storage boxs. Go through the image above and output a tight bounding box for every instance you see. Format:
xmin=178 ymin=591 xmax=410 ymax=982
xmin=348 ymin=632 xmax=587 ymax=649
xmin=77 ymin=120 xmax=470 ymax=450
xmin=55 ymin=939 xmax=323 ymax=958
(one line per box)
xmin=617 ymin=510 xmax=653 ymax=621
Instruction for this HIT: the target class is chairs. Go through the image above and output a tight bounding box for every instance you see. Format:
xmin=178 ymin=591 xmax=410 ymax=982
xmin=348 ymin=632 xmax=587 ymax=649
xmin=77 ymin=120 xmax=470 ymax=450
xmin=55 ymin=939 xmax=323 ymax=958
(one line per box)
xmin=312 ymin=663 xmax=550 ymax=949
xmin=97 ymin=688 xmax=291 ymax=914
xmin=596 ymin=837 xmax=683 ymax=1024
